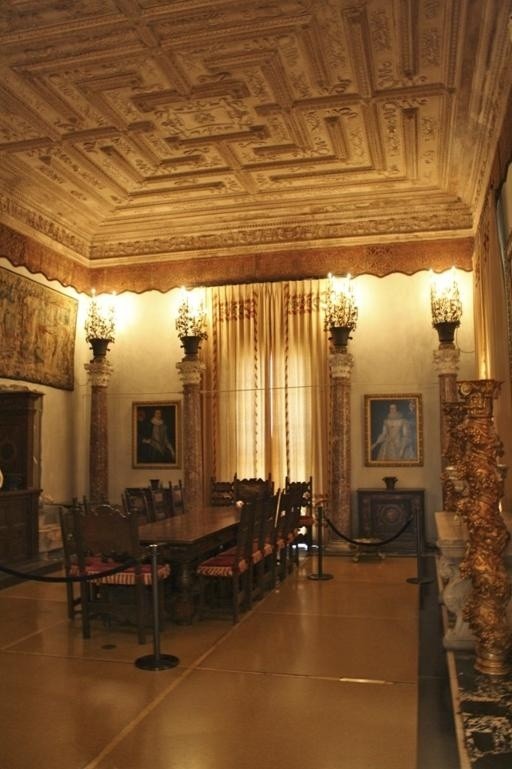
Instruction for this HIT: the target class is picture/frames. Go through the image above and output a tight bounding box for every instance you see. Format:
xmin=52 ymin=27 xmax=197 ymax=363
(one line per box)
xmin=131 ymin=401 xmax=182 ymax=469
xmin=364 ymin=395 xmax=423 ymax=468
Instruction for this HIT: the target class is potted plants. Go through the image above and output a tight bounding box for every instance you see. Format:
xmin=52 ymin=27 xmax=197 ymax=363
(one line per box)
xmin=84 ymin=298 xmax=116 ymax=358
xmin=430 ymin=280 xmax=461 ymax=343
xmin=176 ymin=297 xmax=208 ymax=356
xmin=322 ymin=273 xmax=358 ymax=345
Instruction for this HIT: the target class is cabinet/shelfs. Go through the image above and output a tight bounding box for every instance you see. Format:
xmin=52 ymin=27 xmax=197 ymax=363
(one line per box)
xmin=0 ymin=391 xmax=44 ymax=566
xmin=356 ymin=487 xmax=426 ymax=557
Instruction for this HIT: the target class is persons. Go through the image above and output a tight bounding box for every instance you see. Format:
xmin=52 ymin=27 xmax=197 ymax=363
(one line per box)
xmin=140 ymin=408 xmax=175 ymax=465
xmin=369 ymin=403 xmax=416 ymax=464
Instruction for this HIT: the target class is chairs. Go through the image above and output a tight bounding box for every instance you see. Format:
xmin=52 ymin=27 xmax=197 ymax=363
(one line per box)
xmin=285 ymin=476 xmax=313 ymax=552
xmin=199 ymin=492 xmax=259 ymax=624
xmin=84 ymin=495 xmax=107 ymax=557
xmin=231 ymin=473 xmax=273 ymax=506
xmin=169 ymin=480 xmax=184 ymax=515
xmin=224 ymin=487 xmax=280 ymax=602
xmin=267 ymin=488 xmax=304 ymax=574
xmin=58 ymin=498 xmax=96 ymax=621
xmin=253 ymin=489 xmax=296 ymax=581
xmin=72 ymin=496 xmax=169 ymax=645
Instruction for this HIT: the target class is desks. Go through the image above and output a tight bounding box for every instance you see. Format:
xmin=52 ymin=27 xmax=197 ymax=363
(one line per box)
xmin=138 ymin=506 xmax=241 ymax=625
